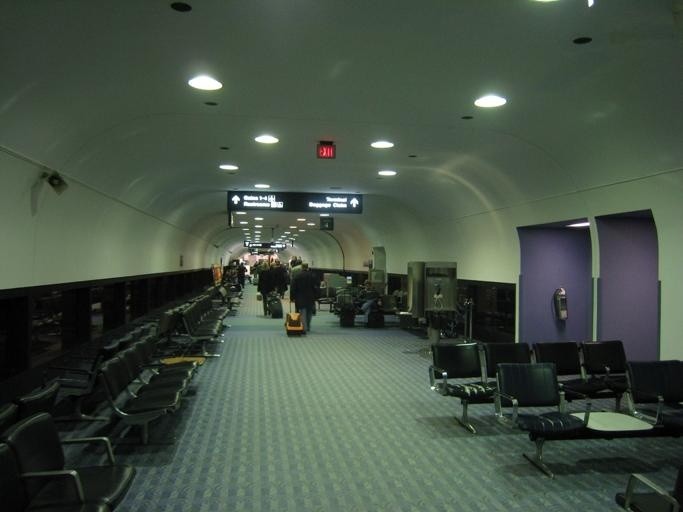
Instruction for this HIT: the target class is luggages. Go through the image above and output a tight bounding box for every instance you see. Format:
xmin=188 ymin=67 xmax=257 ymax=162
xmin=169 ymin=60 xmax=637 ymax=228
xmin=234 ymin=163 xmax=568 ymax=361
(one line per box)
xmin=271 ymin=302 xmax=283 ymax=319
xmin=253 ymin=278 xmax=258 ymax=285
xmin=367 ymin=309 xmax=385 ymax=328
xmin=339 ymin=289 xmax=355 ymax=328
xmin=284 ymin=300 xmax=305 ymax=337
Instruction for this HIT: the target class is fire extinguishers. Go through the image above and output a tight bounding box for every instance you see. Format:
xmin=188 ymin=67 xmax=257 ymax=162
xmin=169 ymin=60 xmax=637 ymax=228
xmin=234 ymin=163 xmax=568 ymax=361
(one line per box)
xmin=554 ymin=288 xmax=567 ymax=320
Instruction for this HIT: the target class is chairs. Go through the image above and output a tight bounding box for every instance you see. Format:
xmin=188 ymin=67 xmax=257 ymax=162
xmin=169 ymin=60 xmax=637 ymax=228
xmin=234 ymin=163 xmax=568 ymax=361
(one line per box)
xmin=0 ymin=411 xmax=137 ymax=512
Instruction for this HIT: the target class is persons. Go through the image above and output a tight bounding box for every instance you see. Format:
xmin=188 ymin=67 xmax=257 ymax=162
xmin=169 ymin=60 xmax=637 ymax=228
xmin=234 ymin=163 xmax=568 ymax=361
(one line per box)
xmin=253 ymin=256 xmax=321 ymax=332
xmin=236 ymin=262 xmax=247 ymax=289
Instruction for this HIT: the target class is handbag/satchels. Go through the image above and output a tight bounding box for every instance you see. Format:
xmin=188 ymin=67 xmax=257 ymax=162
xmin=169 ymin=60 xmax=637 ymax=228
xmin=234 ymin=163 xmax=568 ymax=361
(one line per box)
xmin=256 ymin=291 xmax=263 ymax=301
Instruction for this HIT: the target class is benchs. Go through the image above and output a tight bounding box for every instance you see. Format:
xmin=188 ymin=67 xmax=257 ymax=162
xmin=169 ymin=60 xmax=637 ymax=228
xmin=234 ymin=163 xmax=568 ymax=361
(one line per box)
xmin=493 ymin=360 xmax=681 ymax=480
xmin=318 ymin=281 xmax=413 ymax=329
xmin=615 ymin=454 xmax=681 ymax=510
xmin=429 ymin=340 xmax=627 ymax=434
xmin=131 ymin=294 xmax=231 ymax=357
xmin=0 ymin=321 xmax=206 ymax=445
xmin=203 ymin=277 xmax=245 ymax=312
